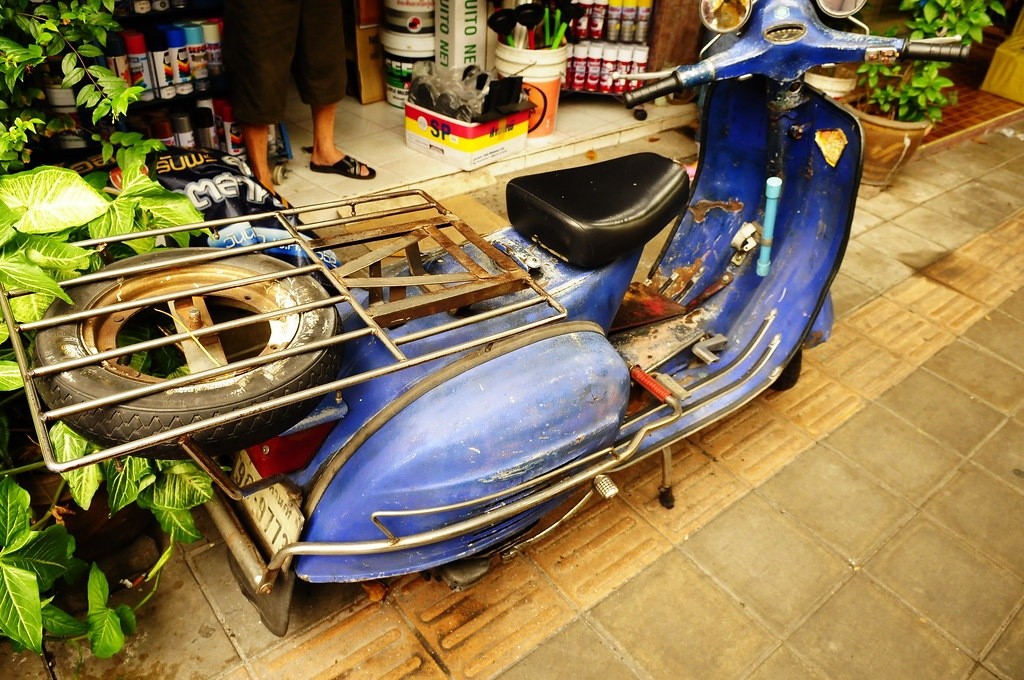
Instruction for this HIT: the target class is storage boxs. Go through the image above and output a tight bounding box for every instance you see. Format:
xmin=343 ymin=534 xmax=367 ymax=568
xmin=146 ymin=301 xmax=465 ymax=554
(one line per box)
xmin=404 ymin=99 xmax=529 ymax=171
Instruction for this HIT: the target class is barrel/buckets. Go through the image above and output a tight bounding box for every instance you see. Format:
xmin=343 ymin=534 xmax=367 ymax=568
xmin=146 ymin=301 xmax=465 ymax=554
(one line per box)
xmin=495 ymin=41 xmax=567 ymax=138
xmin=380 ymin=0 xmax=436 ymax=109
xmin=47 ymin=85 xmax=85 ymax=148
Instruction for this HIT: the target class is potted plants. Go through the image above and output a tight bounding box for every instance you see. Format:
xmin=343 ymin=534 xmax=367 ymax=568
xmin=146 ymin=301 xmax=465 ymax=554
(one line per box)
xmin=0 ymin=165 xmax=230 ymax=680
xmin=843 ymin=0 xmax=1007 ymax=186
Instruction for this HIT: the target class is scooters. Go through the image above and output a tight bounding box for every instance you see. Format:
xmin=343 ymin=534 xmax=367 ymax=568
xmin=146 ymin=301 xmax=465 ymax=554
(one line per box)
xmin=0 ymin=1 xmax=973 ymax=635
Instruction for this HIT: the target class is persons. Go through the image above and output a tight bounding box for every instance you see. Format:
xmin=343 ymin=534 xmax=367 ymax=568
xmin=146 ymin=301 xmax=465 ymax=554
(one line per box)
xmin=221 ymin=0 xmax=376 ymax=221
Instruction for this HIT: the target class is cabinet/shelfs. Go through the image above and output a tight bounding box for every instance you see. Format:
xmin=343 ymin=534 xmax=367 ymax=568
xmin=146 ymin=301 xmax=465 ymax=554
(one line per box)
xmin=92 ymin=0 xmax=293 ymax=186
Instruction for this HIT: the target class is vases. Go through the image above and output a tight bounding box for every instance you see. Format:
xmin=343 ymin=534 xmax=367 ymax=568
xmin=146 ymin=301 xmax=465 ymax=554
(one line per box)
xmin=804 ymin=66 xmax=857 ymax=99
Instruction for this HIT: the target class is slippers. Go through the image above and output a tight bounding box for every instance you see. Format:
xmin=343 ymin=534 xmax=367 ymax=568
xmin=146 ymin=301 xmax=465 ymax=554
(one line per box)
xmin=310 ymin=155 xmax=376 ymax=180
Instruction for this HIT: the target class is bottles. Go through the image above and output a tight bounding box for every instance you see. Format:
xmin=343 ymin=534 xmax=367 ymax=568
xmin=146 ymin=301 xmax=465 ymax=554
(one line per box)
xmin=805 ymin=62 xmax=858 ymax=100
xmin=104 ymin=0 xmax=278 ymax=162
xmin=562 ymin=0 xmax=652 ymax=95
